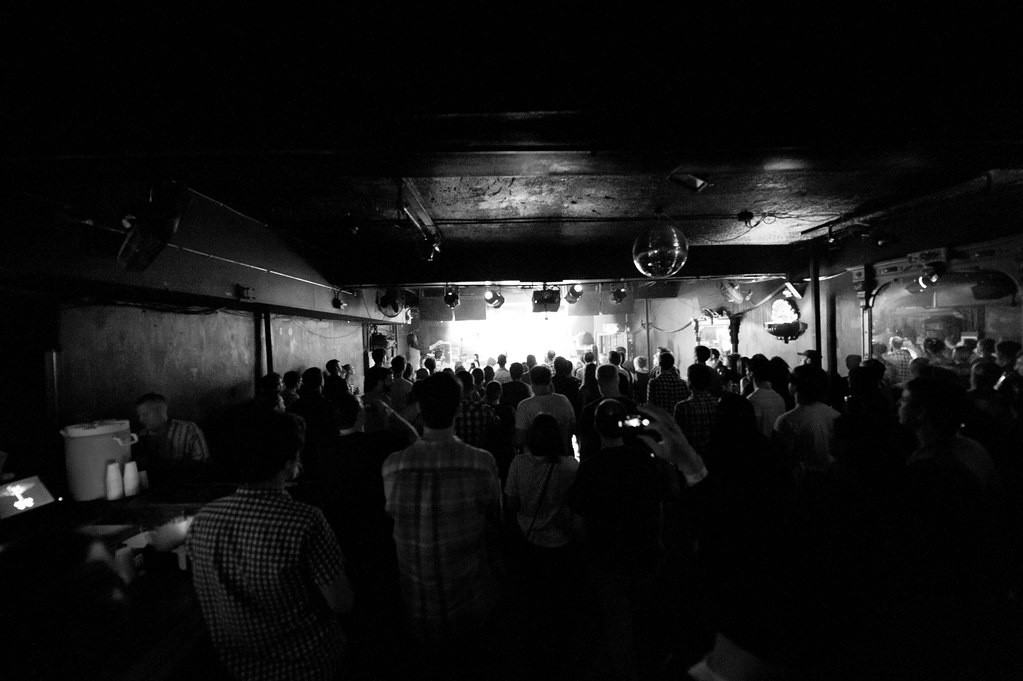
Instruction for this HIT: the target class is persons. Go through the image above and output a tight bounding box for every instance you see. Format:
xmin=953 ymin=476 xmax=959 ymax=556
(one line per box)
xmin=137 ymin=328 xmax=1023 ymax=681
